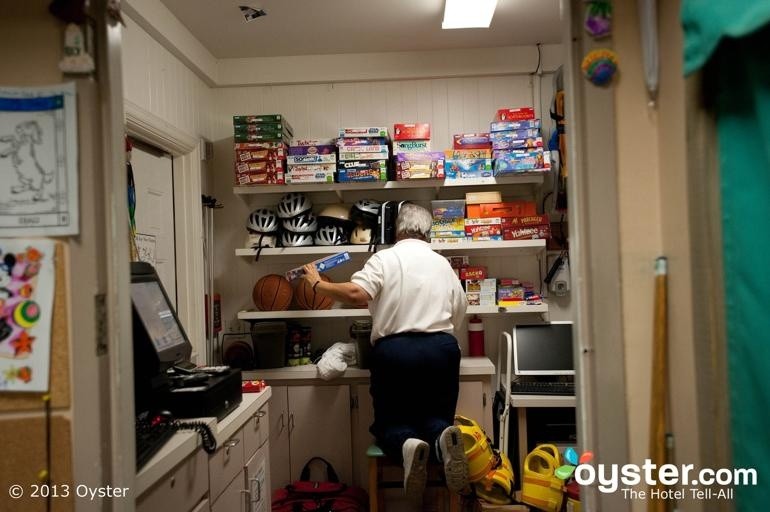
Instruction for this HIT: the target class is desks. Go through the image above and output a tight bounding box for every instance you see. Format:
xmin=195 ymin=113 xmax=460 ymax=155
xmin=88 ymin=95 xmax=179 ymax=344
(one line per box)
xmin=499 ymin=374 xmax=577 ymax=489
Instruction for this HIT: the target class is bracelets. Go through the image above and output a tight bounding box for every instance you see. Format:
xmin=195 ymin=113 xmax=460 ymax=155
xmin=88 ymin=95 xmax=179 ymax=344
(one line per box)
xmin=312 ymin=279 xmax=321 ymax=295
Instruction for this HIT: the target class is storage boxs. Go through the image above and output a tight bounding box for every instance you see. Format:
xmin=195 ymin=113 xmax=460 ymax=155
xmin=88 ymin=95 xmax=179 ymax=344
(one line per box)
xmin=431 ymin=199 xmax=466 ymax=219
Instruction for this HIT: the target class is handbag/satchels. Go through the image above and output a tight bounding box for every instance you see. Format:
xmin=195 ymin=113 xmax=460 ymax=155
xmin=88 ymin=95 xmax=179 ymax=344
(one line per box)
xmin=271 ymin=456 xmax=369 ymax=512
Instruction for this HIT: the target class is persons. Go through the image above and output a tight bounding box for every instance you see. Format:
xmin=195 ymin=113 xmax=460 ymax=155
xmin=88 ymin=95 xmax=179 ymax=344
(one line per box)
xmin=300 ymin=203 xmax=471 ymax=502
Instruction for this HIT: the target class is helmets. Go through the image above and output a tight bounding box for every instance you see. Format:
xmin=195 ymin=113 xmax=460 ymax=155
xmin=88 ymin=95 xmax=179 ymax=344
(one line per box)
xmin=246 ymin=192 xmax=381 ymax=248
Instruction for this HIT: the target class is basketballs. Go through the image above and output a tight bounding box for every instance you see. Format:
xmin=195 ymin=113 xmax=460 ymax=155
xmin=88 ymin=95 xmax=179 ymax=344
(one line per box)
xmin=252 ymin=274 xmax=293 ymax=311
xmin=295 ymin=272 xmax=335 ymax=309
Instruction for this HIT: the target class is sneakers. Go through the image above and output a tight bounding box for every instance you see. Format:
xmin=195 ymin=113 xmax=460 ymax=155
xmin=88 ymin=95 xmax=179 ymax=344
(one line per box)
xmin=402 ymin=438 xmax=429 ymax=502
xmin=438 ymin=427 xmax=469 ymax=490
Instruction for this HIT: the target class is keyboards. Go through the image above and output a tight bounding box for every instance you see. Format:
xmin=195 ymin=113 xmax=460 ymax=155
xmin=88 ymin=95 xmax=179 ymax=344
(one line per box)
xmin=511 ymin=381 xmax=575 ymax=396
xmin=134 ymin=424 xmax=177 ymax=471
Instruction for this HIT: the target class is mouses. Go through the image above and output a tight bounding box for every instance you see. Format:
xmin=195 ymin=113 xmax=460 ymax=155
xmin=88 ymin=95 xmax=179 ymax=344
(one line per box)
xmin=146 ymin=409 xmax=174 ymax=423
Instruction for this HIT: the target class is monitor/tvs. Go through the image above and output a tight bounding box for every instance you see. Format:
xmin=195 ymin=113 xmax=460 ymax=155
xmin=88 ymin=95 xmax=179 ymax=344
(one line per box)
xmin=130 ymin=262 xmax=193 ymax=373
xmin=512 ymin=321 xmax=577 ymax=376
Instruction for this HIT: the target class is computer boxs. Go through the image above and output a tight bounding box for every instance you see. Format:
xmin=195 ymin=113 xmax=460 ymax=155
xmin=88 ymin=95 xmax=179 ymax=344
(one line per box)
xmin=135 ymin=367 xmax=242 ymax=424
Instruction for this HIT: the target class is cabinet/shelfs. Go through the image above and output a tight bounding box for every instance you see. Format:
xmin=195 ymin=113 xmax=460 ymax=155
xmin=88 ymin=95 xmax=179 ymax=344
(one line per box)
xmin=233 ymin=175 xmax=548 ymax=321
xmin=135 ymin=356 xmax=496 ymax=512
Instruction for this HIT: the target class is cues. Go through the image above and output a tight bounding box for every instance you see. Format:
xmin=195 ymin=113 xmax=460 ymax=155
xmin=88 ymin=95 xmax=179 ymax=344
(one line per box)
xmin=649 ymin=257 xmax=677 ymax=511
